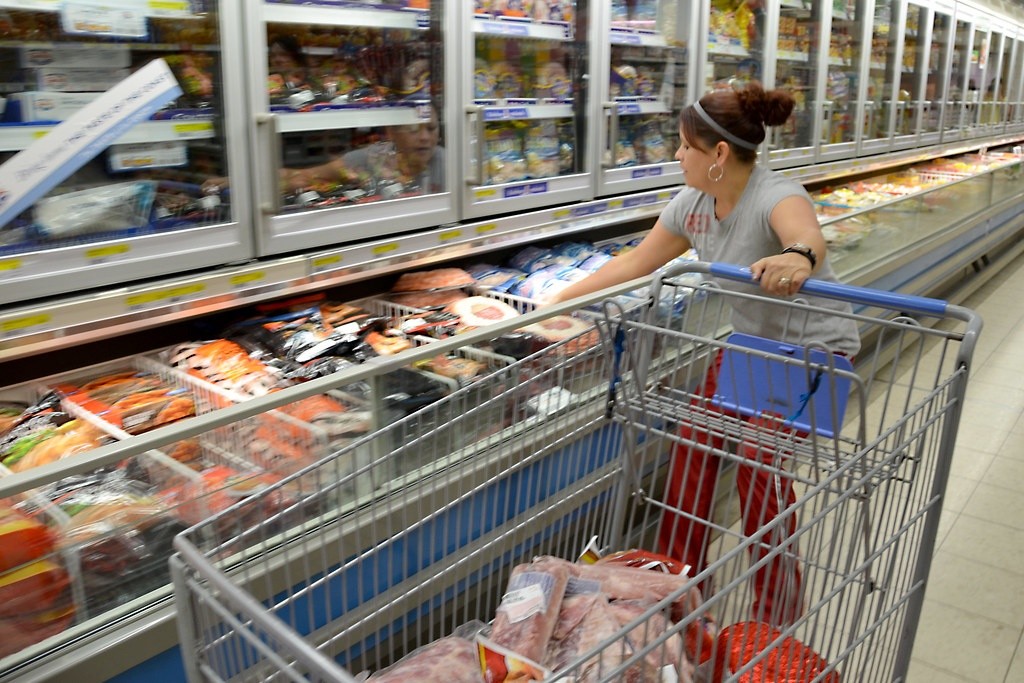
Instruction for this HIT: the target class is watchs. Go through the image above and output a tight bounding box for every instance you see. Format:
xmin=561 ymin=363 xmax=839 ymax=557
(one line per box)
xmin=779 ymin=243 xmax=818 ymax=270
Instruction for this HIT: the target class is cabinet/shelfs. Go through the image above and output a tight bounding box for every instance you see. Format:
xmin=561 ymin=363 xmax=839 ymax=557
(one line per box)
xmin=0 ymin=0 xmax=1024 ymax=311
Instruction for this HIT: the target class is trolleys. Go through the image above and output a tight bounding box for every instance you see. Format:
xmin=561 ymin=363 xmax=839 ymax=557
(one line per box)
xmin=170 ymin=260 xmax=983 ymax=683
xmin=0 ymin=175 xmax=232 ymax=258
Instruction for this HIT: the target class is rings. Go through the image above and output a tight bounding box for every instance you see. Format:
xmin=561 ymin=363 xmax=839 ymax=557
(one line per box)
xmin=778 ymin=277 xmax=792 ymax=285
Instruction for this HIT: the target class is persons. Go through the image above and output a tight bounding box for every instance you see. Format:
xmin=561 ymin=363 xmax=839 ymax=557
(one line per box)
xmin=200 ymin=100 xmax=449 ymax=198
xmin=539 ymin=79 xmax=862 ymax=631
